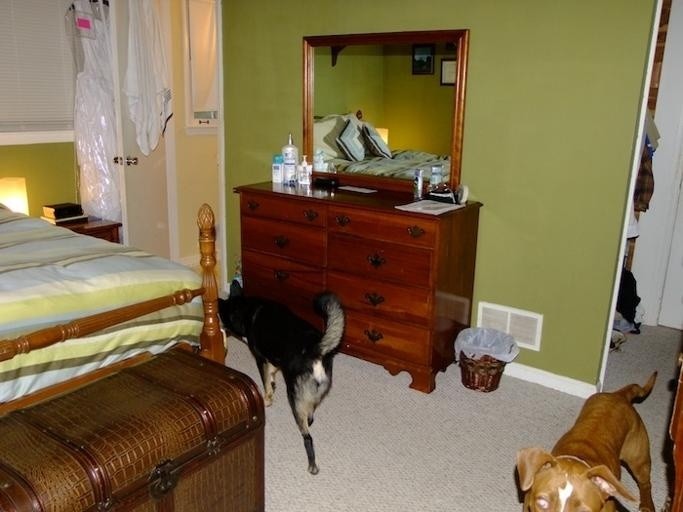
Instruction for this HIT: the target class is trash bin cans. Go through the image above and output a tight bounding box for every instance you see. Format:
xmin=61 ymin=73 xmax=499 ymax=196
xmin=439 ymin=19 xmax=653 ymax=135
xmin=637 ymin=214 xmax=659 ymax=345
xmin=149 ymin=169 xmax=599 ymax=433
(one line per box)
xmin=456 ymin=330 xmax=519 ymax=391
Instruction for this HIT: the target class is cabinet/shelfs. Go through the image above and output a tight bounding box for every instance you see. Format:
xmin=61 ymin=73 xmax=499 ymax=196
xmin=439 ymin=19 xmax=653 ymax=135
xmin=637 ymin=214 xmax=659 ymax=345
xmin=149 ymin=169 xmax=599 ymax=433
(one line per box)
xmin=234 ymin=181 xmax=327 ymax=337
xmin=326 ymin=201 xmax=484 ymax=394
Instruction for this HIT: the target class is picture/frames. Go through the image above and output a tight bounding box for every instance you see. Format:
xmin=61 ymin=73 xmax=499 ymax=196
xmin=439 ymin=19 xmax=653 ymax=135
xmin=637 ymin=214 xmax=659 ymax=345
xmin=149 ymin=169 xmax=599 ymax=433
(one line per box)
xmin=412 ymin=44 xmax=435 ymax=75
xmin=440 ymin=57 xmax=458 ymax=86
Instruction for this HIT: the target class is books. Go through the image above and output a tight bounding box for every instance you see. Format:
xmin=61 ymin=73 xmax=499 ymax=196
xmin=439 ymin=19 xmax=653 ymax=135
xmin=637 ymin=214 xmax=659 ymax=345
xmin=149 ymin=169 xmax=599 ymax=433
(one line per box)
xmin=40 ymin=202 xmax=88 ymax=226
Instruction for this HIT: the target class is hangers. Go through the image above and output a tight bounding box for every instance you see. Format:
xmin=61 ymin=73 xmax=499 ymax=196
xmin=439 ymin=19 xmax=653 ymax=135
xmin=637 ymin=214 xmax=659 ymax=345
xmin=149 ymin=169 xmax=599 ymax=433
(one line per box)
xmin=67 ymin=0 xmax=109 ymax=11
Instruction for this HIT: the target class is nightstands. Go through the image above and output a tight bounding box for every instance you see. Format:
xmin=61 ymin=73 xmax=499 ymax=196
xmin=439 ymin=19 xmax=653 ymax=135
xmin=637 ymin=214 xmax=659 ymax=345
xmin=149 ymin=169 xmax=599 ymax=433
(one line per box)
xmin=64 ymin=214 xmax=123 ymax=244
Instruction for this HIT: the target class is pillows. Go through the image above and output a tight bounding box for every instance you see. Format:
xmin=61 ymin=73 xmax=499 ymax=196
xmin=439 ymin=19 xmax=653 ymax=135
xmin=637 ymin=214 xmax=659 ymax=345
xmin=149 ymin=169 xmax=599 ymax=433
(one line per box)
xmin=313 ymin=116 xmax=347 ymax=162
xmin=0 ymin=203 xmax=27 ymax=224
xmin=363 ymin=125 xmax=392 ymax=159
xmin=335 ymin=120 xmax=367 ymax=162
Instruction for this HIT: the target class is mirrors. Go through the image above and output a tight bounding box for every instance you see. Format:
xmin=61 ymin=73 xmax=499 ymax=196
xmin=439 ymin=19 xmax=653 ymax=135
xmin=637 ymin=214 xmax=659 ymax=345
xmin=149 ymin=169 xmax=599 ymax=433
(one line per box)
xmin=180 ymin=0 xmax=219 ymax=136
xmin=302 ymin=29 xmax=469 ymax=199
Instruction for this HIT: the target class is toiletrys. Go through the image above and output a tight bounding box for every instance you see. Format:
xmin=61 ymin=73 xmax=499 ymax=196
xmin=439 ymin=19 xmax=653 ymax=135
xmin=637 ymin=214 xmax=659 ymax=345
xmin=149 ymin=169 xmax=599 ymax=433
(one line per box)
xmin=431 ymin=164 xmax=442 ymax=183
xmin=413 ymin=167 xmax=423 ymax=198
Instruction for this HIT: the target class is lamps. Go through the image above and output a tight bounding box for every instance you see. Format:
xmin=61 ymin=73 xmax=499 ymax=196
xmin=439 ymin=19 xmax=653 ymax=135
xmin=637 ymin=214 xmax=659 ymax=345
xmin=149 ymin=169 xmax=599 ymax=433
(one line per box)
xmin=0 ymin=177 xmax=29 ymax=217
xmin=374 ymin=128 xmax=389 ymax=145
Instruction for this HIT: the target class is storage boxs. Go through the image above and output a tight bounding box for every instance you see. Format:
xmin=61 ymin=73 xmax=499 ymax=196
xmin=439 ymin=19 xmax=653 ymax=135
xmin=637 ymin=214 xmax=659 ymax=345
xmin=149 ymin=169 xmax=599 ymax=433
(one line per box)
xmin=0 ymin=346 xmax=266 ymax=512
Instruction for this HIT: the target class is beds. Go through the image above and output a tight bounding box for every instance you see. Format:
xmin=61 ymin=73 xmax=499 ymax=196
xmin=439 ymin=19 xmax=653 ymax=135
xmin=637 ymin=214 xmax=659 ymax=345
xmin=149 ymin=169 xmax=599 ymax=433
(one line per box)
xmin=0 ymin=142 xmax=228 ymax=415
xmin=313 ymin=113 xmax=452 ymax=182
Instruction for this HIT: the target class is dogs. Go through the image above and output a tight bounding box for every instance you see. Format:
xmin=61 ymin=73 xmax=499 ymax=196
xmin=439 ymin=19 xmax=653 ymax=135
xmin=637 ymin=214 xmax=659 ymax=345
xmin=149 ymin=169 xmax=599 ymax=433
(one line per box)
xmin=218 ymin=280 xmax=346 ymax=475
xmin=517 ymin=370 xmax=659 ymax=512
